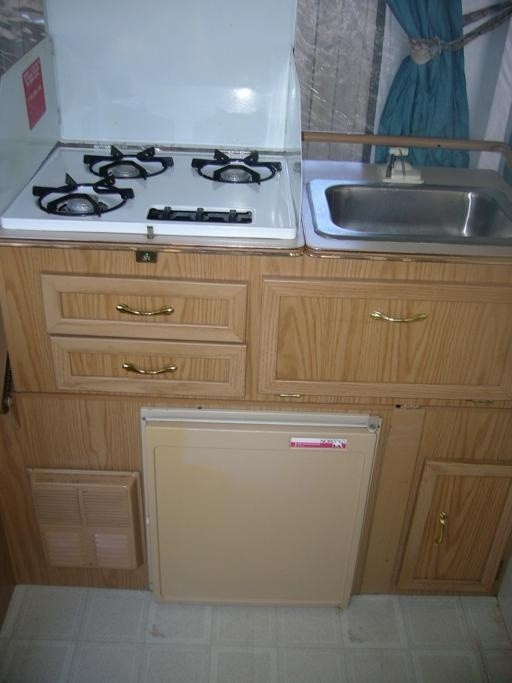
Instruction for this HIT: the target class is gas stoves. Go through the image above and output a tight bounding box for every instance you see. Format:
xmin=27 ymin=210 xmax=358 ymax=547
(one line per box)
xmin=1 ymin=141 xmax=300 ymax=242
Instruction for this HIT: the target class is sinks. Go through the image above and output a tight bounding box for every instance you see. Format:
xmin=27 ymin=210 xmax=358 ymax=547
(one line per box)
xmin=306 ymin=176 xmax=512 ymax=245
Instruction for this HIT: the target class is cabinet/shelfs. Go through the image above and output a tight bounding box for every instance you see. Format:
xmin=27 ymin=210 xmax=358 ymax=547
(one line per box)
xmin=8 ymin=392 xmax=150 ymax=598
xmin=392 ymin=406 xmax=510 ymax=598
xmin=4 ymin=241 xmax=262 ymax=400
xmin=262 ymin=254 xmax=511 ymax=401
